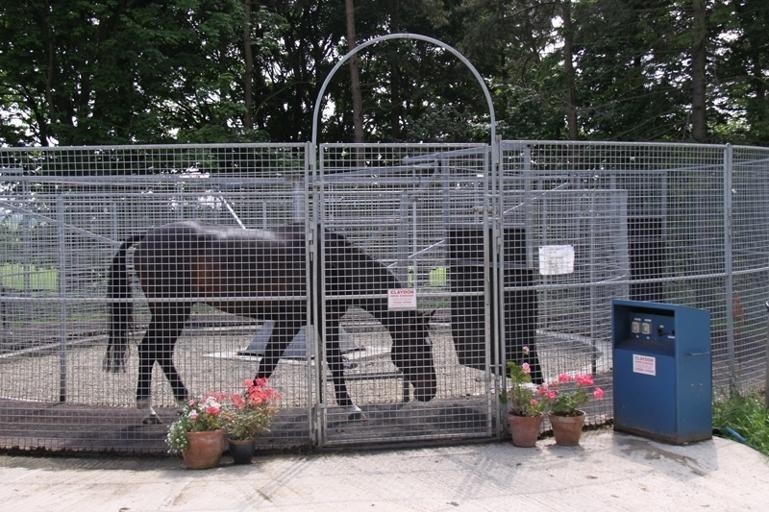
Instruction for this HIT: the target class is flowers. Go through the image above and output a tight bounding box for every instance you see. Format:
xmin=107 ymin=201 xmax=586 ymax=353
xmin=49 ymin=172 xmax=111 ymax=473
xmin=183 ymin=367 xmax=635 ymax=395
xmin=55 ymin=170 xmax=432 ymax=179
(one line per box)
xmin=549 ymin=373 xmax=604 ymax=414
xmin=160 ymin=391 xmax=223 ymax=455
xmin=498 ymin=346 xmax=559 ymax=416
xmin=222 ymin=377 xmax=282 ymax=439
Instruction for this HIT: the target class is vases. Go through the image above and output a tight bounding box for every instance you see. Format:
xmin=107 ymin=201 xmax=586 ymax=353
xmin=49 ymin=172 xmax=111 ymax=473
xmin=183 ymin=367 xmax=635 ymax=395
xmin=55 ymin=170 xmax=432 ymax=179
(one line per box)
xmin=508 ymin=411 xmax=544 ymax=447
xmin=547 ymin=410 xmax=587 ymax=446
xmin=181 ymin=428 xmax=224 ymax=468
xmin=227 ymin=439 xmax=256 ymax=463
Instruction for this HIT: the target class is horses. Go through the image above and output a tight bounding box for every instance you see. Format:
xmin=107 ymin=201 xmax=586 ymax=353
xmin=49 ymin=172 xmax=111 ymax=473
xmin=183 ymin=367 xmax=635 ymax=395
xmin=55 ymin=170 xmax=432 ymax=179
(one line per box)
xmin=100 ymin=220 xmax=438 ymax=428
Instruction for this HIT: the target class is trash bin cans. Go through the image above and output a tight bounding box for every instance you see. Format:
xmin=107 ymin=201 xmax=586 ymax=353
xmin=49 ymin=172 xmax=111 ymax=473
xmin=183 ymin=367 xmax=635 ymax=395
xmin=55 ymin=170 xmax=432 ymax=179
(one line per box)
xmin=612 ymin=298 xmax=713 ymax=445
